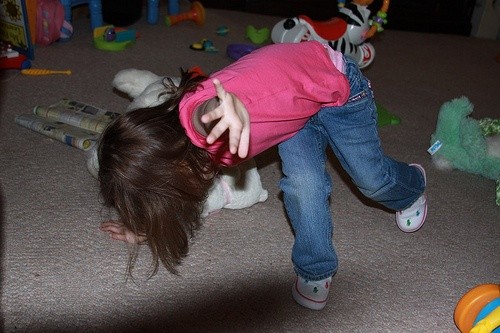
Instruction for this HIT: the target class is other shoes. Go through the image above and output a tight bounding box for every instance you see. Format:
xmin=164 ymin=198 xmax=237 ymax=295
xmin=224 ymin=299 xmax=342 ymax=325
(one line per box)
xmin=292 ymin=273 xmax=332 ymax=310
xmin=396 ymin=163 xmax=428 ymax=232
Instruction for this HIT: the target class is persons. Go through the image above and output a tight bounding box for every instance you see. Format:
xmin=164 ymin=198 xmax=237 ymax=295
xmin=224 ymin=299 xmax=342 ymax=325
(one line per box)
xmin=96 ymin=41 xmax=428 ymax=311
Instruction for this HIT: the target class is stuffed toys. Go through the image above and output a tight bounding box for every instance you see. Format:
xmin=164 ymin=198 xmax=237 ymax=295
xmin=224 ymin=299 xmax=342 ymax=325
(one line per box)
xmin=87 ymin=68 xmax=269 ymax=218
xmin=429 ymin=96 xmax=500 ymax=206
